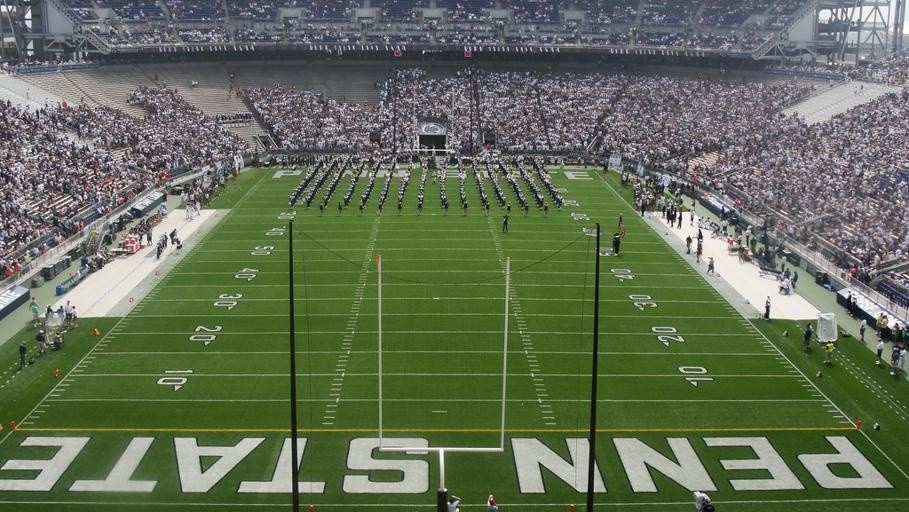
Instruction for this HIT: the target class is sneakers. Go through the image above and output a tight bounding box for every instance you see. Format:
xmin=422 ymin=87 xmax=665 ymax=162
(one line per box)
xmin=889 ymin=371 xmax=897 ymax=376
xmin=823 ymin=359 xmax=831 ymax=364
xmin=764 ymin=316 xmax=770 ymax=321
xmin=875 ymin=360 xmax=882 ymax=366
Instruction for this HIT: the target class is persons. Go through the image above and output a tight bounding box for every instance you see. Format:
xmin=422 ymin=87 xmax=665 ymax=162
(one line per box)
xmin=471 ymin=1 xmax=908 ymax=371
xmin=447 ymin=494 xmax=462 ymax=511
xmin=855 ymin=418 xmax=862 ymax=429
xmin=2 ymin=1 xmax=909 ymax=371
xmin=487 ymin=494 xmax=498 ymax=511
xmin=694 ymin=491 xmax=715 ymax=511
